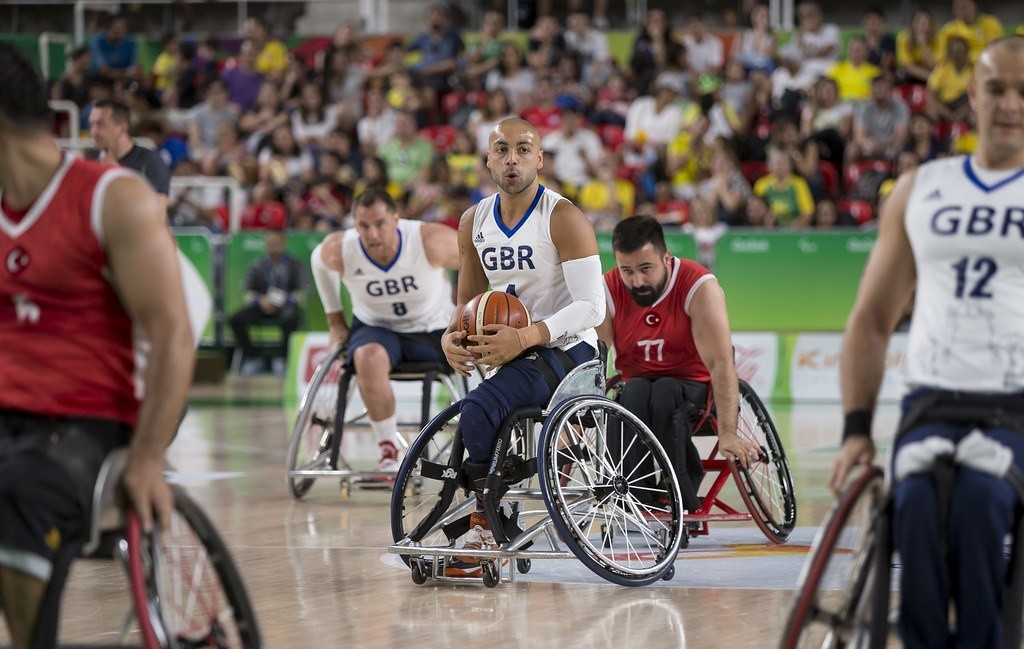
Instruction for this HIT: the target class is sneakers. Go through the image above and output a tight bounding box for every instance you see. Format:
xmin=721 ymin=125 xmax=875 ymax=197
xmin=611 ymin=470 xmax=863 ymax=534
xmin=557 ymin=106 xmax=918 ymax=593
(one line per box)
xmin=444 ymin=511 xmax=500 ymax=577
xmin=355 ymin=440 xmax=404 ymax=489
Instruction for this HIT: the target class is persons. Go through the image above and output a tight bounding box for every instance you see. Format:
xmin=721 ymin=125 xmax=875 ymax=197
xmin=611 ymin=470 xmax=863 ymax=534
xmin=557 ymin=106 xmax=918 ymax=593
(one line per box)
xmin=308 ymin=185 xmax=461 ymax=491
xmin=831 ymin=35 xmax=1024 ymax=649
xmin=225 ymin=229 xmax=309 ymax=375
xmin=594 ymin=214 xmax=764 ymax=539
xmin=48 ymin=0 xmax=1024 ymax=229
xmin=440 ymin=117 xmax=607 ymax=577
xmin=0 ymin=40 xmax=198 ymax=649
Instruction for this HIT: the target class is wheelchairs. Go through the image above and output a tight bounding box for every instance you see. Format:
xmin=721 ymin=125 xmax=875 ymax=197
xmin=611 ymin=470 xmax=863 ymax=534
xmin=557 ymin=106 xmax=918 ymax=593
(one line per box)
xmin=30 ymin=447 xmax=269 ymax=649
xmin=556 ymin=344 xmax=798 ymax=545
xmin=284 ymin=338 xmax=527 ymax=500
xmin=388 ymin=395 xmax=685 ymax=592
xmin=774 ymin=467 xmax=1024 ymax=649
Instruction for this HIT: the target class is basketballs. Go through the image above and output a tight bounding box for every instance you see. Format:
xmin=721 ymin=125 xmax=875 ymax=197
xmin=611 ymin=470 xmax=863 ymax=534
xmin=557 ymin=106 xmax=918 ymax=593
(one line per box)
xmin=455 ymin=288 xmax=533 ymax=367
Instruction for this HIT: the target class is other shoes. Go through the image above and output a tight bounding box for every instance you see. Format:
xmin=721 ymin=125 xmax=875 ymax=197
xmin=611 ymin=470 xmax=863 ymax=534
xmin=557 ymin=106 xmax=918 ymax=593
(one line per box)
xmin=243 ymin=355 xmax=264 ymax=377
xmin=271 ymin=357 xmax=286 ymax=377
